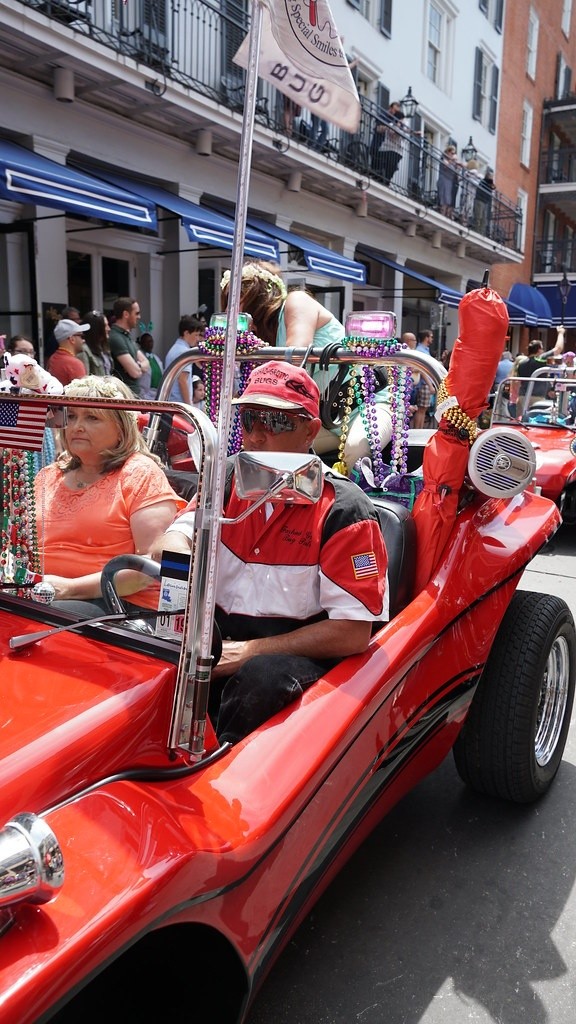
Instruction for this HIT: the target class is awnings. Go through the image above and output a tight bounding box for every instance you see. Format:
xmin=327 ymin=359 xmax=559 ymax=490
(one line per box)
xmin=509 ymin=283 xmax=552 ymax=328
xmin=0 ymin=140 xmax=280 ymax=261
xmin=356 ymin=247 xmax=465 ymax=309
xmin=201 ymin=197 xmax=366 ymax=286
xmin=503 ymin=300 xmax=538 ymax=328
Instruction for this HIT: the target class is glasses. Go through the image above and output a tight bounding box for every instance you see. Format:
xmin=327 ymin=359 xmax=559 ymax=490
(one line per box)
xmin=92 ymin=309 xmax=103 ymax=321
xmin=74 ymin=334 xmax=84 ymax=339
xmin=240 ymin=408 xmax=311 ymax=434
xmin=12 ymin=348 xmax=36 ymax=356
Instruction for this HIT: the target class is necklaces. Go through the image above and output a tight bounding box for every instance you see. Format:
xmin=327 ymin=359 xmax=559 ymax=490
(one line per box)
xmin=338 ymin=337 xmax=478 ymax=491
xmin=74 ymin=467 xmax=103 ymax=488
xmin=0 ymin=448 xmax=41 ymax=599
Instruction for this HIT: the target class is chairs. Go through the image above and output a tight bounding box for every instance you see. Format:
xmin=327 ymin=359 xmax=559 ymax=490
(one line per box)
xmin=299 ymin=121 xmax=337 ymax=152
xmin=368 ymin=496 xmax=417 ymax=633
xmin=411 ymin=182 xmax=438 ymax=206
xmin=35 ymin=0 xmax=90 ymax=24
xmin=116 ymin=29 xmax=170 ymax=72
xmin=221 ymin=76 xmax=269 ymax=124
xmin=163 ymin=470 xmax=198 ymax=501
xmin=347 ymin=142 xmax=380 ymax=174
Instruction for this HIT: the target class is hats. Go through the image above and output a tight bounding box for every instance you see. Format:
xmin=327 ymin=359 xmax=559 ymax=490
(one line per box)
xmin=231 ymin=360 xmax=320 ymax=418
xmin=53 ymin=319 xmax=90 ymax=339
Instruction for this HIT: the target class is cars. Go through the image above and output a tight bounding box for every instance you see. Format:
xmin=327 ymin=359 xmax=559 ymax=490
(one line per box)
xmin=484 ymin=365 xmax=576 ymax=529
xmin=0 ymin=351 xmax=576 ymax=1023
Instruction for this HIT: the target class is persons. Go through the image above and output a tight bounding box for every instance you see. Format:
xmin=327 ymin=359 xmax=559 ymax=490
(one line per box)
xmin=0 ymin=296 xmax=164 ymax=394
xmin=439 ymin=321 xmax=576 ymax=422
xmin=414 ymin=329 xmax=435 ymax=428
xmin=191 ymin=313 xmax=207 ymax=381
xmin=371 ymin=102 xmax=421 ymax=188
xmin=154 ymin=360 xmax=389 ymax=748
xmin=221 ymin=262 xmax=405 ymax=477
xmin=192 ymin=375 xmax=206 ymax=411
xmin=166 ymin=315 xmax=205 ymax=406
xmin=403 ymin=332 xmax=420 ymax=405
xmin=281 ymin=56 xmax=359 ymax=146
xmin=24 ymin=376 xmax=189 ymax=618
xmin=437 ymin=146 xmax=496 ymax=235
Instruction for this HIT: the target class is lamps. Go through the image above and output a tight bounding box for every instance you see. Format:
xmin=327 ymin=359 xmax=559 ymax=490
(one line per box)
xmin=405 ymin=223 xmax=416 ymax=238
xmin=402 ymin=86 xmax=419 ymax=117
xmin=456 ymin=244 xmax=466 ymax=260
xmin=461 ymin=136 xmax=477 ymax=161
xmin=431 ymin=231 xmax=442 ymax=248
xmin=286 ymin=172 xmax=302 ymax=191
xmin=193 ymin=130 xmax=212 ymax=157
xmin=355 ymin=201 xmax=368 ymax=218
xmin=54 ymin=67 xmax=76 ymax=106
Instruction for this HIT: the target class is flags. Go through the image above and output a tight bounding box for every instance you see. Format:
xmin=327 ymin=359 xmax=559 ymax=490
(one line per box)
xmin=233 ymin=0 xmax=362 ymax=134
